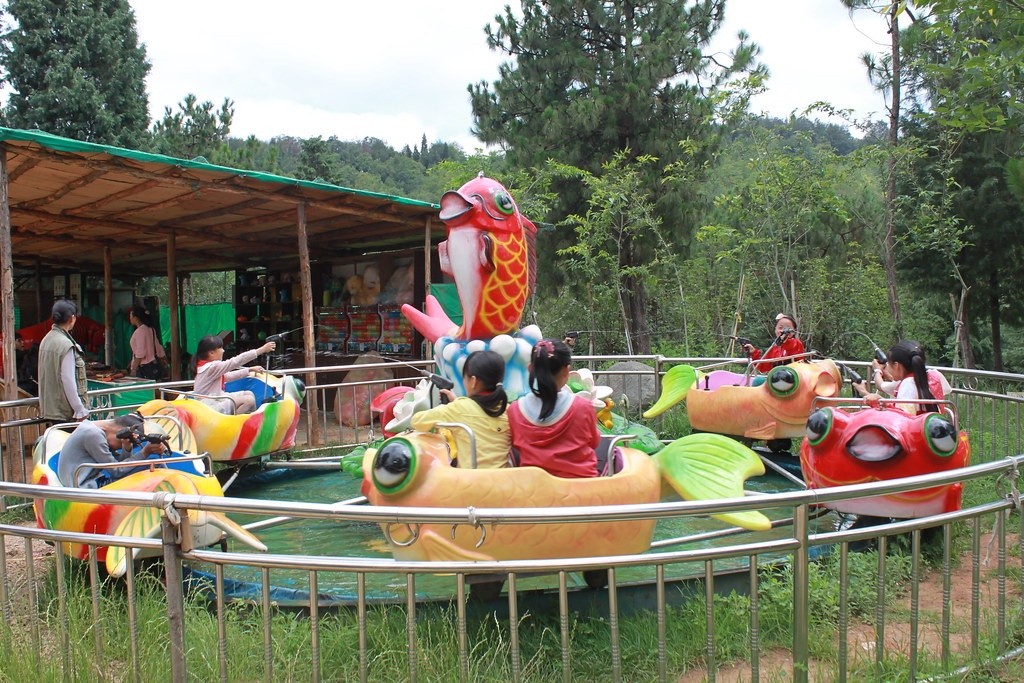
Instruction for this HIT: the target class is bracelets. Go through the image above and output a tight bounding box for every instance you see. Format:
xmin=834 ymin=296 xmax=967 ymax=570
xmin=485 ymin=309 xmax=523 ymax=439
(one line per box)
xmin=132 ymin=368 xmax=136 ymax=370
xmin=873 ymin=369 xmax=883 ymax=375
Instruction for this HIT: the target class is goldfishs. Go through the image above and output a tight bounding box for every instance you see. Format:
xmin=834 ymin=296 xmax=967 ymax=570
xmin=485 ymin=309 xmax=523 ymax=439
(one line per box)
xmin=24 ymin=368 xmax=308 ymax=574
xmin=636 ymin=359 xmax=970 ymax=525
xmin=357 ymin=435 xmax=771 ymax=575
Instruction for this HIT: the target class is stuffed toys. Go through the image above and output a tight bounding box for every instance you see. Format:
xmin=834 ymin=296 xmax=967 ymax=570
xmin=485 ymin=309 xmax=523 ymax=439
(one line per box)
xmin=319 ymin=261 xmax=415 ymax=304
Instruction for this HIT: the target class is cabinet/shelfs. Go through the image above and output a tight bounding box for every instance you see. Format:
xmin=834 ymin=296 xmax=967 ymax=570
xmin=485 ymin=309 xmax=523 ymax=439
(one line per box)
xmin=231 ymin=284 xmax=298 ymax=353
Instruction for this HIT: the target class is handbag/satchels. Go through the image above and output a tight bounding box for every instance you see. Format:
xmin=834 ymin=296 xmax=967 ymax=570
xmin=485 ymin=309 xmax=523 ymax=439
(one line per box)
xmin=139 ymin=358 xmax=168 ymax=381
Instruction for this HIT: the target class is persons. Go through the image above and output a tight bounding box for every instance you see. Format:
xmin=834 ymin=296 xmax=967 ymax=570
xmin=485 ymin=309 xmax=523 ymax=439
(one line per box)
xmin=58 ymin=415 xmax=169 ymax=489
xmin=130 ymin=308 xmax=167 ymax=380
xmin=740 ymin=314 xmax=806 ymax=387
xmin=507 ymin=337 xmax=599 ymax=479
xmin=15 ymin=332 xmax=38 ymax=398
xmin=37 ymin=300 xmax=88 ymax=434
xmin=194 ymin=337 xmax=276 ymax=414
xmin=411 ymin=351 xmax=512 ymax=469
xmin=852 ymin=340 xmax=952 ymax=415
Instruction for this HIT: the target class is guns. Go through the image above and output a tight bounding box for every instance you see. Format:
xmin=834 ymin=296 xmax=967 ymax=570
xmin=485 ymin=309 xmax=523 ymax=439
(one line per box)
xmin=775 ymin=328 xmax=793 ymax=346
xmin=265 ymin=330 xmax=288 ymax=352
xmin=116 ymin=423 xmax=139 ymax=445
xmin=565 ymin=330 xmax=582 ymax=346
xmin=872 ymin=342 xmax=888 ymax=364
xmin=841 ymin=363 xmax=862 ymax=388
xmin=141 ymin=433 xmax=172 ymax=456
xmin=419 ymin=368 xmax=455 ymax=404
xmin=727 ymin=334 xmax=751 ymax=353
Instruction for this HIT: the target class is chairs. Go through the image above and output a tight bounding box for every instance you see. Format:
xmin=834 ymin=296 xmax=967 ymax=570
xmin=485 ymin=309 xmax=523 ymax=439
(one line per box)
xmin=0 ymin=378 xmax=46 ymax=447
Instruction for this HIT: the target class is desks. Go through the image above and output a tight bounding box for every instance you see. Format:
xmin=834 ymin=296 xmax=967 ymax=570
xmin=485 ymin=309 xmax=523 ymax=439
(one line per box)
xmin=87 ymin=375 xmax=156 ymax=422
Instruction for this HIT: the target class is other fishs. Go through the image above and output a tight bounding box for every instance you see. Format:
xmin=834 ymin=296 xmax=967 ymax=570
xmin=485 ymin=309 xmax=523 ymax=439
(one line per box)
xmin=399 ymin=169 xmax=536 ymax=345
